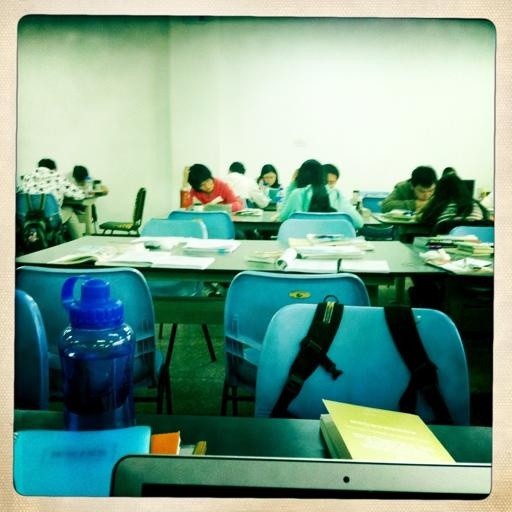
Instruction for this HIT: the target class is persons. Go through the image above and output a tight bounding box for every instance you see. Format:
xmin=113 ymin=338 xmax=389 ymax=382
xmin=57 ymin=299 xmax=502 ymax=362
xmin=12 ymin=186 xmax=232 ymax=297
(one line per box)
xmin=61 ymin=166 xmax=109 ymax=225
xmin=221 ymin=162 xmax=272 ymax=209
xmin=180 ymin=163 xmax=243 ymax=213
xmin=413 ymin=173 xmax=488 ymax=235
xmin=380 ymin=166 xmax=438 ymax=214
xmin=442 ymin=167 xmax=455 ymax=178
xmin=256 ymin=163 xmax=280 ymax=188
xmin=321 ymin=164 xmax=340 ymax=200
xmin=16 ymin=158 xmax=89 ymax=240
xmin=279 ymin=159 xmax=365 ymax=230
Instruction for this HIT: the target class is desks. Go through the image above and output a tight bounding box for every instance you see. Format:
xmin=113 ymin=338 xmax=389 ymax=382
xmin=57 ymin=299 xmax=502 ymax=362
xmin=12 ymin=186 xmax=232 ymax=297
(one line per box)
xmin=14 ymin=408 xmax=492 ymax=464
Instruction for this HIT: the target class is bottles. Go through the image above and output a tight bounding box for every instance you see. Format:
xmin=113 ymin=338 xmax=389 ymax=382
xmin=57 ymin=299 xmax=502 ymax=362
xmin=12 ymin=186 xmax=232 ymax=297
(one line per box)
xmin=352 ymin=190 xmax=360 ymax=206
xmin=275 ymin=188 xmax=286 ymax=213
xmin=84 ymin=176 xmax=92 ymax=192
xmin=56 ymin=273 xmax=138 ymax=432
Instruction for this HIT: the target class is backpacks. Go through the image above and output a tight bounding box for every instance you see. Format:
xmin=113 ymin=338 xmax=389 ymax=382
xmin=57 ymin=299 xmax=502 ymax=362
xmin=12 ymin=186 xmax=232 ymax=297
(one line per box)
xmin=21 ymin=193 xmax=62 ymax=254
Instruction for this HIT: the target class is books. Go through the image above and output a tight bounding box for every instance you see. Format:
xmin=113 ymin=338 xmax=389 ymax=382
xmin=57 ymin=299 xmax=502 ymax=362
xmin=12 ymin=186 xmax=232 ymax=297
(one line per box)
xmin=379 ymin=207 xmax=418 ymax=222
xmin=187 ymin=201 xmax=263 ymax=216
xmin=244 ymin=232 xmax=390 ymax=272
xmin=414 ymin=235 xmax=494 ymax=275
xmin=48 ymin=234 xmax=240 ymax=270
xmin=318 ymin=398 xmax=457 ymax=465
xmin=13 ymin=425 xmax=207 ymax=497
